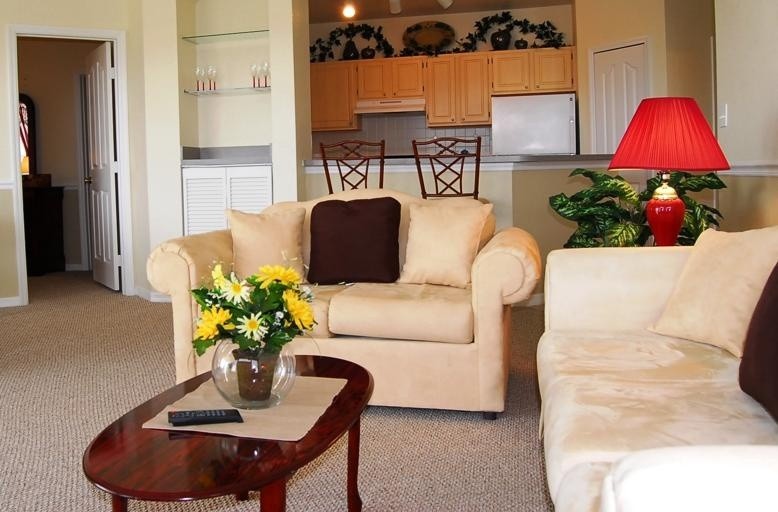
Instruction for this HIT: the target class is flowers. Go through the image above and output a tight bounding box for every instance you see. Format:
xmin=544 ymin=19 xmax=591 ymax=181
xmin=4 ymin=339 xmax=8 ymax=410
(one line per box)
xmin=186 ymin=248 xmax=332 ymax=357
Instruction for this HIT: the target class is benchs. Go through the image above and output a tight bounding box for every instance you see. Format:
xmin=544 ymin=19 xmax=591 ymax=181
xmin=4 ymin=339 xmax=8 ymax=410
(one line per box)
xmin=142 ymin=186 xmax=544 ymax=423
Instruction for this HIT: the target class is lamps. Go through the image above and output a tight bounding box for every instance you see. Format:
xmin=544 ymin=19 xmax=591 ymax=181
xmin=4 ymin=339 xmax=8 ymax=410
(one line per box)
xmin=601 ymin=92 xmax=734 ymax=247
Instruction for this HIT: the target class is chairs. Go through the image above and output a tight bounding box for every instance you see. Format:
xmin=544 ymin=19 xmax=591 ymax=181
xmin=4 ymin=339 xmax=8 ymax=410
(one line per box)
xmin=410 ymin=134 xmax=483 ymax=200
xmin=318 ymin=137 xmax=385 ymax=196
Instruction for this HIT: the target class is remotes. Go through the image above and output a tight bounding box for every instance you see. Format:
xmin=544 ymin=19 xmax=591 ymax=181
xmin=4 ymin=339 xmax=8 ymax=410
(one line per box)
xmin=167 ymin=408 xmax=244 ymax=426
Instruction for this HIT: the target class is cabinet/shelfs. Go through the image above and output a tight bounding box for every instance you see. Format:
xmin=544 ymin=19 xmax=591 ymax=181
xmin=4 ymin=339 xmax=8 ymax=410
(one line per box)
xmin=180 ymin=161 xmax=278 ymax=239
xmin=181 ymin=28 xmax=271 ymax=99
xmin=309 ymin=56 xmax=362 ymax=133
xmin=422 ymin=50 xmax=495 ymax=129
xmin=350 ymin=53 xmax=429 ymax=118
xmin=489 ymin=43 xmax=578 ymax=97
xmin=19 ymin=184 xmax=68 ymax=280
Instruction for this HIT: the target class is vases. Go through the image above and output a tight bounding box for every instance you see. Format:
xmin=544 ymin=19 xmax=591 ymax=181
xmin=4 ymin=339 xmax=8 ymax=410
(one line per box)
xmin=209 ymin=337 xmax=300 ymax=413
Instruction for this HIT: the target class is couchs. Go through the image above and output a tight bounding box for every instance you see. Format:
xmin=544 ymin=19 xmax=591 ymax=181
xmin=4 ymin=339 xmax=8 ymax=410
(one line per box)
xmin=533 ymin=219 xmax=778 ymax=511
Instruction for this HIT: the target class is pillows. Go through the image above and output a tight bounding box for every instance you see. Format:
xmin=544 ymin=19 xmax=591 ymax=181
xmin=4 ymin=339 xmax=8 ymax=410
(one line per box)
xmin=222 ymin=201 xmax=309 ymax=286
xmin=649 ymin=222 xmax=777 ymax=359
xmin=305 ymin=193 xmax=403 ymax=285
xmin=736 ymin=256 xmax=777 ymax=429
xmin=395 ymin=198 xmax=493 ymax=292
